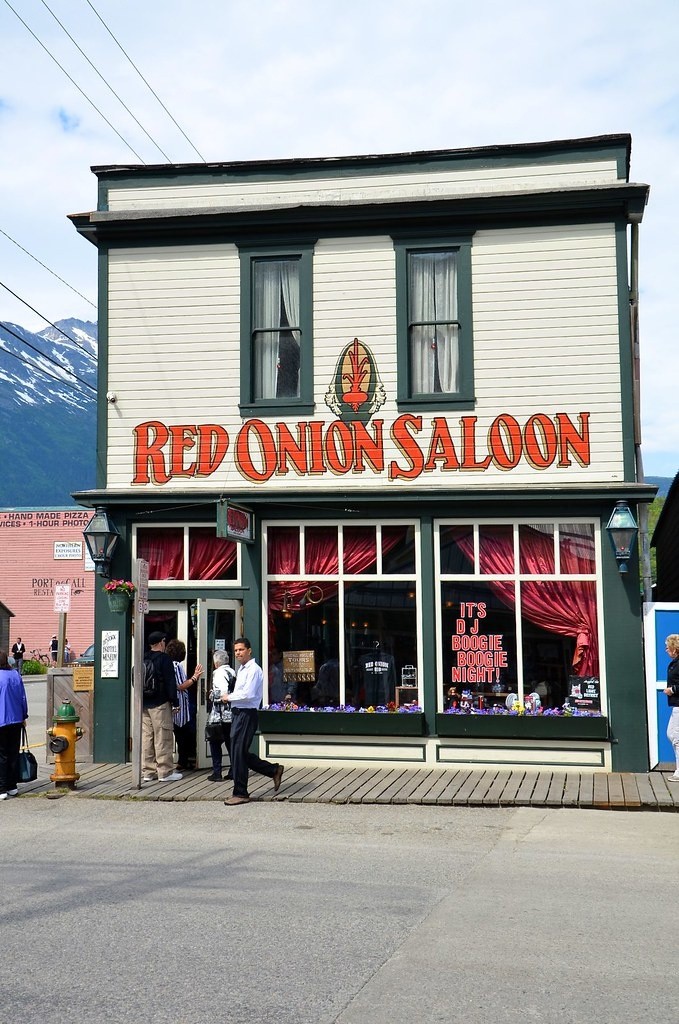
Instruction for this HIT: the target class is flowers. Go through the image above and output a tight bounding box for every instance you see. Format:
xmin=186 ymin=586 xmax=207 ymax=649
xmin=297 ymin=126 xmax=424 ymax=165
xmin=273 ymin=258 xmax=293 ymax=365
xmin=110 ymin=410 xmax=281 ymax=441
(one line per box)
xmin=100 ymin=579 xmax=138 ymax=598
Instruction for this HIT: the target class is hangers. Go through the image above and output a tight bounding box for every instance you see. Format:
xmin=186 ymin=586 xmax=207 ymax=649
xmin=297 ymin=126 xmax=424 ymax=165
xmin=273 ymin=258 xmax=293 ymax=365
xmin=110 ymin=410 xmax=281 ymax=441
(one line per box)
xmin=371 ymin=641 xmax=381 ymax=655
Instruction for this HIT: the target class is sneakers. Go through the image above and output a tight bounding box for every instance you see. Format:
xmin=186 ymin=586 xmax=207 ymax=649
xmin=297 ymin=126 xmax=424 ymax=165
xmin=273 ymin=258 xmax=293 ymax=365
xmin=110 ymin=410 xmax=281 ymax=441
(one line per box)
xmin=144 ymin=773 xmax=158 ymax=781
xmin=158 ymin=772 xmax=183 ymax=781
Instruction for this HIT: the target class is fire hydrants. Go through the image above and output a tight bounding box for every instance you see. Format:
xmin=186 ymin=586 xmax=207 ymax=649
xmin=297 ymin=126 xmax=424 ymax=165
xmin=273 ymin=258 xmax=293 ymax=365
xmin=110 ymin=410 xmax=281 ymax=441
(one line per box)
xmin=46 ymin=697 xmax=85 ymax=789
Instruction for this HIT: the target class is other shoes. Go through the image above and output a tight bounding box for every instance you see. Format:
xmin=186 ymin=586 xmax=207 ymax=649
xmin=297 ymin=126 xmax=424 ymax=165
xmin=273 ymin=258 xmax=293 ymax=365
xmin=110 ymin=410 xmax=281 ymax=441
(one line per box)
xmin=7 ymin=788 xmax=19 ymax=796
xmin=207 ymin=773 xmax=222 ymax=781
xmin=667 ymin=770 xmax=679 ymax=783
xmin=273 ymin=765 xmax=284 ymax=791
xmin=224 ymin=774 xmax=234 ymax=780
xmin=0 ymin=792 xmax=8 ymax=800
xmin=175 ymin=763 xmax=196 ymax=771
xmin=224 ymin=795 xmax=250 ymax=804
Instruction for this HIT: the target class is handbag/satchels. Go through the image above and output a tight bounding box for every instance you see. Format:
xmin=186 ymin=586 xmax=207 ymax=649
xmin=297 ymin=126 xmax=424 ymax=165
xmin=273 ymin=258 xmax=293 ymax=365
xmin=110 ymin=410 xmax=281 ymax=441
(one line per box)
xmin=207 ymin=723 xmax=225 ymax=736
xmin=17 ymin=725 xmax=37 ymax=783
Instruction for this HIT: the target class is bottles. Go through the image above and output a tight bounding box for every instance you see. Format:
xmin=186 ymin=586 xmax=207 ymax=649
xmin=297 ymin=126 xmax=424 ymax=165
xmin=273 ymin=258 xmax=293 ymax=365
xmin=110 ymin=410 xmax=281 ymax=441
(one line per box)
xmin=479 ymin=695 xmax=484 ymax=710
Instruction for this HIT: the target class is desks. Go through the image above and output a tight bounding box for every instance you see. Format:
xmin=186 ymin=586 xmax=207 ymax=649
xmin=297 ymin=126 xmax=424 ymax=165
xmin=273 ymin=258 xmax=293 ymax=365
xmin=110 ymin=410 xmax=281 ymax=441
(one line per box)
xmin=395 ymin=686 xmax=418 ymax=708
xmin=471 ymin=692 xmax=548 ymax=709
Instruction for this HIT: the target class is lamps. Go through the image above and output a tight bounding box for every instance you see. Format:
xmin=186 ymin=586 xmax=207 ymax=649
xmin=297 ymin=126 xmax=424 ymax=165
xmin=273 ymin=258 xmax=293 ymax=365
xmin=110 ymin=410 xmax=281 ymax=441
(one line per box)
xmin=81 ymin=506 xmax=122 ymax=578
xmin=606 ymin=499 xmax=640 ymax=573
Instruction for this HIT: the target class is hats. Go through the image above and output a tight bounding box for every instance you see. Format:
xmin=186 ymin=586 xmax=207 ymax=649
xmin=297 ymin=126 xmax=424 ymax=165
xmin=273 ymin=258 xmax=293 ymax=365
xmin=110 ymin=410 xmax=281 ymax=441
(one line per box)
xmin=148 ymin=631 xmax=167 ymax=644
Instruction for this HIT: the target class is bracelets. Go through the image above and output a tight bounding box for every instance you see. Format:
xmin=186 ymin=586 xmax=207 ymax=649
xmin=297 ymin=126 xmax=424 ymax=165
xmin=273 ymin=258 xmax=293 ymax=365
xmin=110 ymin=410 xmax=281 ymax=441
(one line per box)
xmin=192 ymin=676 xmax=197 ymax=682
xmin=172 ymin=706 xmax=181 ymax=712
xmin=671 ymin=688 xmax=674 ymax=694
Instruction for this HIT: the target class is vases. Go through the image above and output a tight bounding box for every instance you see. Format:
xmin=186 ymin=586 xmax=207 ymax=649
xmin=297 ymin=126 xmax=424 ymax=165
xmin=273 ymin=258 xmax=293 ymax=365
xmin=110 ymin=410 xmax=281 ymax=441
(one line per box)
xmin=107 ymin=592 xmax=130 ymax=612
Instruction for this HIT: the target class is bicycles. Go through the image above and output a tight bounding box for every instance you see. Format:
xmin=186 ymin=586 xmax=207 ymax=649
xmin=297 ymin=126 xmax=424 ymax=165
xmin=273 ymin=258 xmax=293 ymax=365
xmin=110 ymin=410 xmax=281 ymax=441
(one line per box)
xmin=30 ymin=648 xmax=50 ymax=667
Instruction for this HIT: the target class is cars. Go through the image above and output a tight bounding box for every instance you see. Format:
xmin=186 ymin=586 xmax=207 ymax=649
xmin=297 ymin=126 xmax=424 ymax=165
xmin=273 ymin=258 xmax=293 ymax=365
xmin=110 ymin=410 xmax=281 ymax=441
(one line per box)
xmin=72 ymin=644 xmax=94 ymax=663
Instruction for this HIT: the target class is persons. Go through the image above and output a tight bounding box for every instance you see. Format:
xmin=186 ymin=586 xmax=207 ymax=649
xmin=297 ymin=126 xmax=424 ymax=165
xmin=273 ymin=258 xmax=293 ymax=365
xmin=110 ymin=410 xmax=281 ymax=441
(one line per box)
xmin=218 ymin=638 xmax=286 ymax=805
xmin=269 ymin=642 xmax=339 ymax=707
xmin=0 ymin=651 xmax=29 ymax=799
xmin=48 ymin=635 xmax=58 ymax=662
xmin=64 ymin=639 xmax=70 ymax=662
xmin=207 ymin=648 xmax=236 ymax=783
xmin=165 ymin=639 xmax=207 ymax=769
xmin=130 ymin=633 xmax=183 ymax=781
xmin=11 ymin=637 xmax=25 ymax=674
xmin=664 ymin=634 xmax=679 ymax=782
